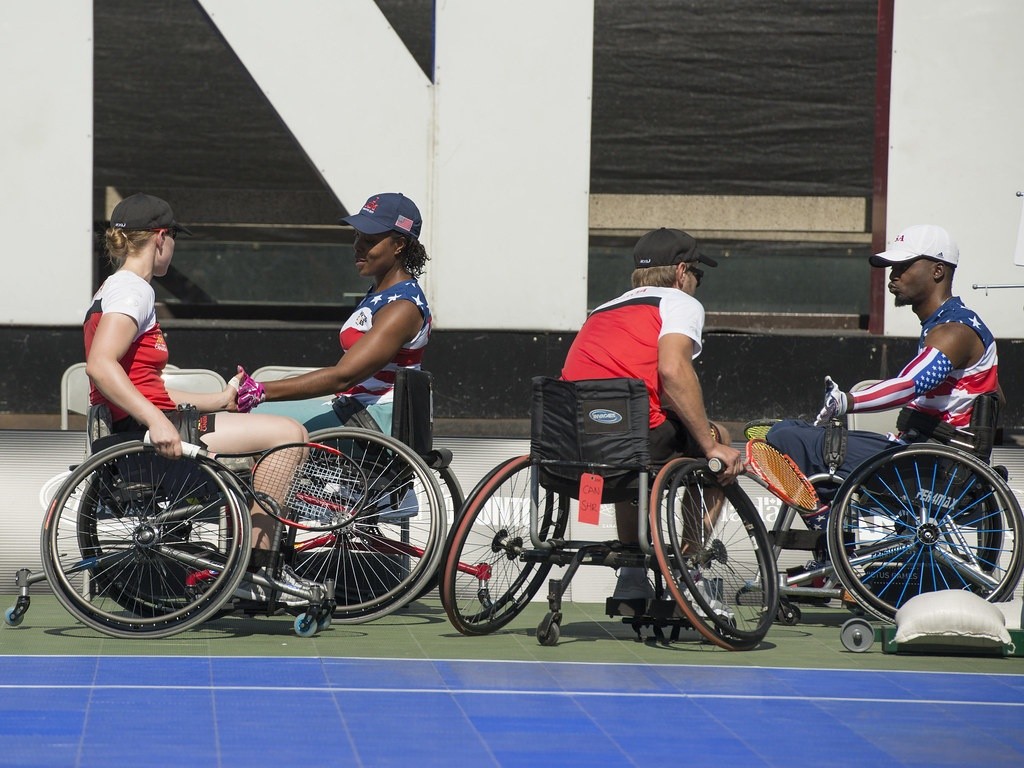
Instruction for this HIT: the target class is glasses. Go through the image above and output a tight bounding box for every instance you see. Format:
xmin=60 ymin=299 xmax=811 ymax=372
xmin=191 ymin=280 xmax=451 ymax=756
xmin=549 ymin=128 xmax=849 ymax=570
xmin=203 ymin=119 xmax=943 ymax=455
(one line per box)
xmin=688 ymin=266 xmax=704 ymax=287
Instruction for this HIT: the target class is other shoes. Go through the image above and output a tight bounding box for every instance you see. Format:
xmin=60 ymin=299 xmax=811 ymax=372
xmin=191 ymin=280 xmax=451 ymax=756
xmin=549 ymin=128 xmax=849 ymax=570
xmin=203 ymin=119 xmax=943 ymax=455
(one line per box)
xmin=786 ymin=552 xmax=866 ymax=589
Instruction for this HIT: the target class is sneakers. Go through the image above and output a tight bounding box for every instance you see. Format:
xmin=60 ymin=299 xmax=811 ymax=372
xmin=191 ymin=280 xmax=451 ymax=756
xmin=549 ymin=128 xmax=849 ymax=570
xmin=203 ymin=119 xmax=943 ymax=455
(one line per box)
xmin=233 ymin=560 xmax=327 ymax=606
xmin=612 ymin=575 xmax=672 ymax=606
xmin=662 ymin=573 xmax=734 ymax=618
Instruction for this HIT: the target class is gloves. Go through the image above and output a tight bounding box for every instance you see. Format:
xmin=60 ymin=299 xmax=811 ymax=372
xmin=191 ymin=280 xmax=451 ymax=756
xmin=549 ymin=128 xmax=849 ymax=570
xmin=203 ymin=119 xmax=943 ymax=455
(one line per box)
xmin=237 ymin=365 xmax=266 ymax=412
xmin=813 ymin=375 xmax=848 ymax=428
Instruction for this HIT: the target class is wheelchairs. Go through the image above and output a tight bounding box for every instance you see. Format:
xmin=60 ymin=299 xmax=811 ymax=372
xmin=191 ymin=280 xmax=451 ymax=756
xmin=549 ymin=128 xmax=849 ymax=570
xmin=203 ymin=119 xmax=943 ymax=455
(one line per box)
xmin=3 ymin=403 xmax=338 ymax=638
xmin=439 ymin=376 xmax=780 ymax=651
xmin=736 ymin=393 xmax=1024 ymax=625
xmin=184 ymin=367 xmax=464 ymax=624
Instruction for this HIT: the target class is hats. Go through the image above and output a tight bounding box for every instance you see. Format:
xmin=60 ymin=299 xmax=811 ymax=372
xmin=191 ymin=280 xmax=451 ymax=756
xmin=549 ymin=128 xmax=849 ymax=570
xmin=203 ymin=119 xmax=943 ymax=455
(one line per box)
xmin=633 ymin=227 xmax=718 ymax=268
xmin=869 ymin=225 xmax=959 ymax=268
xmin=337 ymin=192 xmax=422 ymax=239
xmin=110 ymin=192 xmax=193 ymax=239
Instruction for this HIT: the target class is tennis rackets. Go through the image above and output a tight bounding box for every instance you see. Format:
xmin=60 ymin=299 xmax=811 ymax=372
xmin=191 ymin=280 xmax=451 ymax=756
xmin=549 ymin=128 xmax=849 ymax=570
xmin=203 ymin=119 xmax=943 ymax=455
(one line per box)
xmin=706 ymin=437 xmax=822 ymax=516
xmin=742 ymin=417 xmax=789 ymax=443
xmin=142 ymin=426 xmax=372 ymax=534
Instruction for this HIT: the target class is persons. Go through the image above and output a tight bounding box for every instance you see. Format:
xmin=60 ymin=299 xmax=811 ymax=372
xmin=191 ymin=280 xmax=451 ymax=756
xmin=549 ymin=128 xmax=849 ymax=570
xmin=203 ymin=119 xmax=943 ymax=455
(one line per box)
xmin=560 ymin=228 xmax=744 ymax=620
xmin=84 ymin=193 xmax=328 ymax=606
xmin=764 ymin=223 xmax=1006 ymax=604
xmin=237 ymin=192 xmax=432 ymax=479
